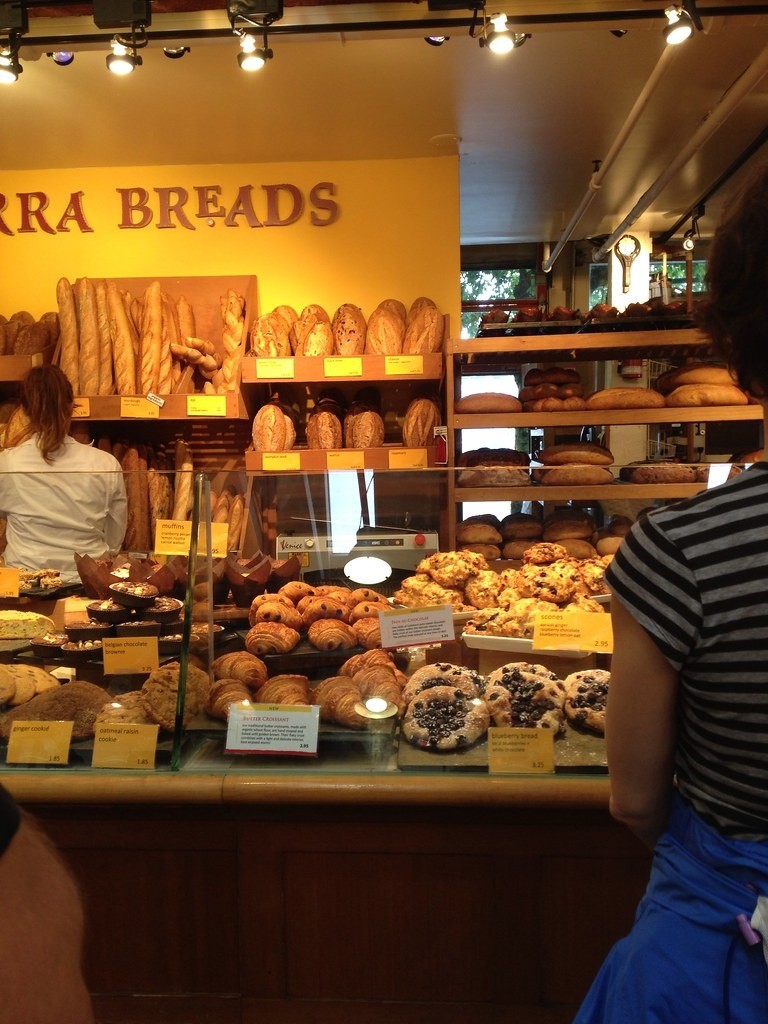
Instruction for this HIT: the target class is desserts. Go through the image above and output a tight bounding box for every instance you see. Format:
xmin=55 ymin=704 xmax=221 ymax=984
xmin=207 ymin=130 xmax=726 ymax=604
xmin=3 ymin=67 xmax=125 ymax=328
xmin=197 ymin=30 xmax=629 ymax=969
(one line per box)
xmin=29 ymin=580 xmax=224 ymax=661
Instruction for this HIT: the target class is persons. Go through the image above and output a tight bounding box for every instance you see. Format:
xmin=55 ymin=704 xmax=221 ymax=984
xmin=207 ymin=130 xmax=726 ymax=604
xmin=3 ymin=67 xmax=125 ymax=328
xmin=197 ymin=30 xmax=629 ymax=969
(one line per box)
xmin=0 ymin=364 xmax=129 ymax=601
xmin=572 ymin=170 xmax=768 ymax=1024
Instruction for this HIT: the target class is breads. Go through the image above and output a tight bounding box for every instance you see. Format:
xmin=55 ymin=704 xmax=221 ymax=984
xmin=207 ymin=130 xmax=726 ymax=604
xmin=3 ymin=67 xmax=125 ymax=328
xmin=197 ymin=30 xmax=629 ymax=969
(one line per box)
xmin=251 ymin=385 xmax=442 ymax=453
xmin=92 ymin=435 xmax=245 ymax=557
xmin=0 ymin=609 xmax=212 ymax=744
xmin=456 ymin=298 xmax=764 ymax=558
xmin=209 ymin=541 xmax=619 ymax=749
xmin=56 ymin=276 xmax=245 ymax=396
xmin=0 ymin=311 xmax=59 ymax=356
xmin=251 ymin=295 xmax=446 ymax=357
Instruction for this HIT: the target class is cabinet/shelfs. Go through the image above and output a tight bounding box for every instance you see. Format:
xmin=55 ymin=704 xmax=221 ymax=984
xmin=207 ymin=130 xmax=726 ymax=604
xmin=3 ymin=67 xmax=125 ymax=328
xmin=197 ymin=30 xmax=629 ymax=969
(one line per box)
xmin=0 ymin=275 xmax=768 ymax=586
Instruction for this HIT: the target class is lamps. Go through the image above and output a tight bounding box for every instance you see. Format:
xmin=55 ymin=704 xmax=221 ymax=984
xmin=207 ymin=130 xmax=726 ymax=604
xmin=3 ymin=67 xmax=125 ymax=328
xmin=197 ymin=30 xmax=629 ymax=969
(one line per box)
xmin=163 ymin=46 xmax=190 ymax=59
xmin=478 ymin=12 xmax=529 ymax=55
xmin=46 ymin=52 xmax=75 ymax=66
xmin=0 ymin=49 xmax=24 ymax=85
xmin=105 ymin=33 xmax=143 ymax=75
xmin=663 ymin=5 xmax=694 ymax=46
xmin=236 ymin=35 xmax=274 ymax=72
xmin=684 ymin=236 xmax=698 ymax=252
xmin=424 ymin=36 xmax=451 ymax=47
